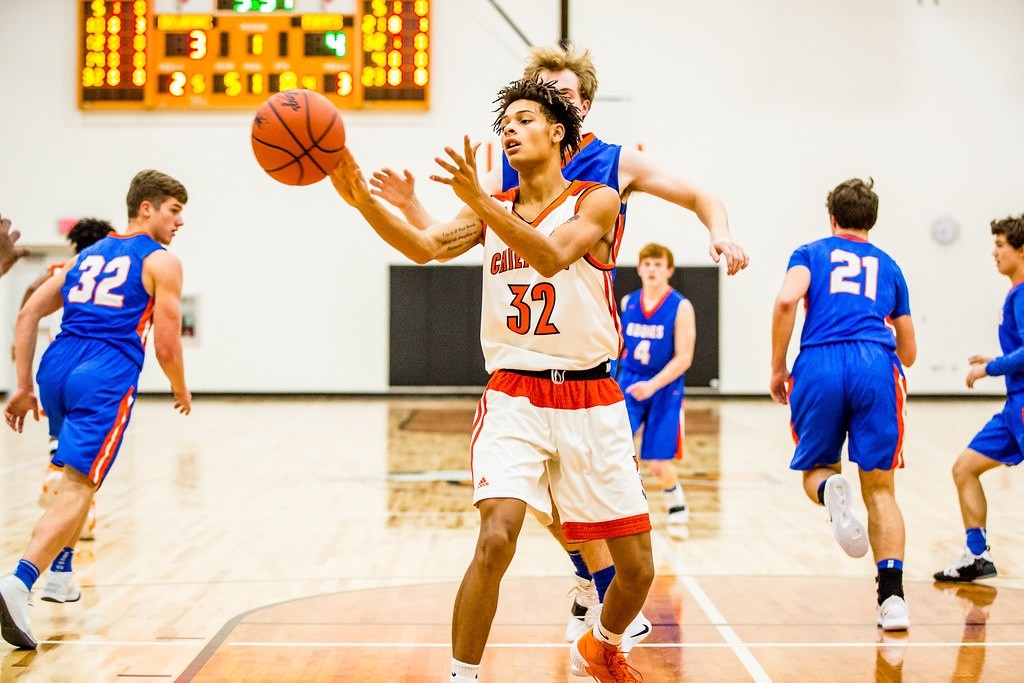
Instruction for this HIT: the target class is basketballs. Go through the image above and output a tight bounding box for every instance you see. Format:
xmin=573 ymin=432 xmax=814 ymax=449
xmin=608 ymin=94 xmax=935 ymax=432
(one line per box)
xmin=251 ymin=88 xmax=346 ymax=187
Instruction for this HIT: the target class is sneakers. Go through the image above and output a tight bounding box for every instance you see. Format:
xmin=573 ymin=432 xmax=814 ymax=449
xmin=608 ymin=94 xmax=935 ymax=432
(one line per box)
xmin=0 ymin=574 xmax=39 ymax=650
xmin=79 ymin=501 xmax=97 ymax=540
xmin=37 ymin=465 xmax=64 ymax=508
xmin=875 ymin=594 xmax=910 ymax=630
xmin=571 ymin=603 xmax=652 ymax=677
xmin=41 ymin=569 xmax=81 ymax=603
xmin=823 ymin=473 xmax=868 ymax=558
xmin=564 ymin=571 xmax=600 ymax=642
xmin=933 ymin=545 xmax=997 ymax=582
xmin=662 ymin=482 xmax=688 ymax=524
xmin=570 ymin=627 xmax=646 ymax=683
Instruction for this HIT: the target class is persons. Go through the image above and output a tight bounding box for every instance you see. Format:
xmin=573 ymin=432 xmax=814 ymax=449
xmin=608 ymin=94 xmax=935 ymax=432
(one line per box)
xmin=617 ymin=244 xmax=697 ymax=518
xmin=767 ymin=176 xmax=917 ymax=630
xmin=874 ymin=624 xmax=909 ymax=683
xmin=329 ymin=75 xmax=656 ymax=682
xmin=0 ymin=167 xmax=192 ymax=648
xmin=369 ymin=43 xmax=750 ymax=640
xmin=932 ymin=214 xmax=1024 ymax=581
xmin=0 ymin=215 xmax=117 ymax=540
xmin=935 ymin=581 xmax=997 ymax=683
